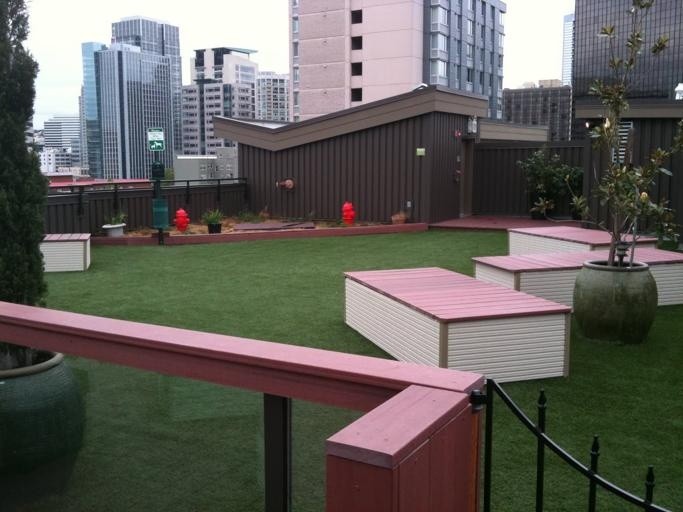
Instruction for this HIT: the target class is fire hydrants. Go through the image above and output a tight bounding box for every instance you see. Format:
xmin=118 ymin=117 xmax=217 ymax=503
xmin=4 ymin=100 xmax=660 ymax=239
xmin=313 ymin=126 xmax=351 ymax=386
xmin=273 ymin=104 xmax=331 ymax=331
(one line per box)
xmin=341 ymin=201 xmax=355 ymax=224
xmin=172 ymin=208 xmax=190 ymax=231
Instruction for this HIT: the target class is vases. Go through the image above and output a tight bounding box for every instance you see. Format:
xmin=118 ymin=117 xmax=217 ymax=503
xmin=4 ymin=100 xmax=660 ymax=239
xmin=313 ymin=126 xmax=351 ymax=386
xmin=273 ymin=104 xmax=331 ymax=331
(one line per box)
xmin=575 ymin=260 xmax=657 ymax=345
xmin=0 ymin=352 xmax=85 ymax=505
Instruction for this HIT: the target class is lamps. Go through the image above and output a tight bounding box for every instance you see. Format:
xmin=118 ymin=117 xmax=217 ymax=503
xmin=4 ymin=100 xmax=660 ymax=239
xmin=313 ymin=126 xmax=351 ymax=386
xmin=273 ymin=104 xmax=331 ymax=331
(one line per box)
xmin=471 ymin=114 xmax=479 ymax=135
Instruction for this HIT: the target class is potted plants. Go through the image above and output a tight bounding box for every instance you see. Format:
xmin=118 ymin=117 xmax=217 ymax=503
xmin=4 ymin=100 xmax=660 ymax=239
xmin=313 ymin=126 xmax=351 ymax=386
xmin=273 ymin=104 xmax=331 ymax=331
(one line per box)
xmin=515 ymin=149 xmax=553 ymax=222
xmin=555 ymin=163 xmax=585 ymax=220
xmin=202 ymin=209 xmax=223 ymax=233
xmin=101 ymin=212 xmax=127 ymax=236
